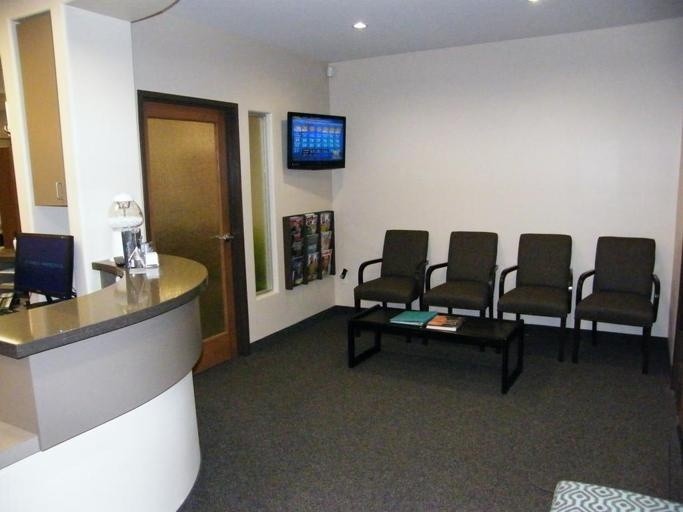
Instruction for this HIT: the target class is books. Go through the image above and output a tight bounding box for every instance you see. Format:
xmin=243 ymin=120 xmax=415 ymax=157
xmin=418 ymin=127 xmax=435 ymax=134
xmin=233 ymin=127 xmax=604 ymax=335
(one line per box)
xmin=289 ymin=212 xmax=332 ymax=286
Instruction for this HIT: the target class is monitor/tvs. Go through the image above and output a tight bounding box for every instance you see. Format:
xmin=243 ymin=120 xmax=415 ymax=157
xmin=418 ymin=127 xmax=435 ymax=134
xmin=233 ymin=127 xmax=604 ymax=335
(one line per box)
xmin=14 ymin=233 xmax=74 ymax=309
xmin=287 ymin=112 xmax=346 ymax=170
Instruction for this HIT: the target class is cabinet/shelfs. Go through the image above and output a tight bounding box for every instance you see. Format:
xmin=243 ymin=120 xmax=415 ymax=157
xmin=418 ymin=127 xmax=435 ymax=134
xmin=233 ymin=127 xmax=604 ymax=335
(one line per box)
xmin=12 ymin=9 xmax=70 ymax=209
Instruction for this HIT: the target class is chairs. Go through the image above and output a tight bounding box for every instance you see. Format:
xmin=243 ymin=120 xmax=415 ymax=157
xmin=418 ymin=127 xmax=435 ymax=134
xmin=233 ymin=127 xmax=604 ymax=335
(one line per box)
xmin=350 ymin=225 xmax=424 ymax=344
xmin=420 ymin=231 xmax=497 ymax=351
xmin=492 ymin=232 xmax=574 ymax=365
xmin=570 ymin=236 xmax=664 ymax=374
xmin=546 ymin=478 xmax=681 ymax=512
xmin=4 ymin=232 xmax=74 ymax=308
